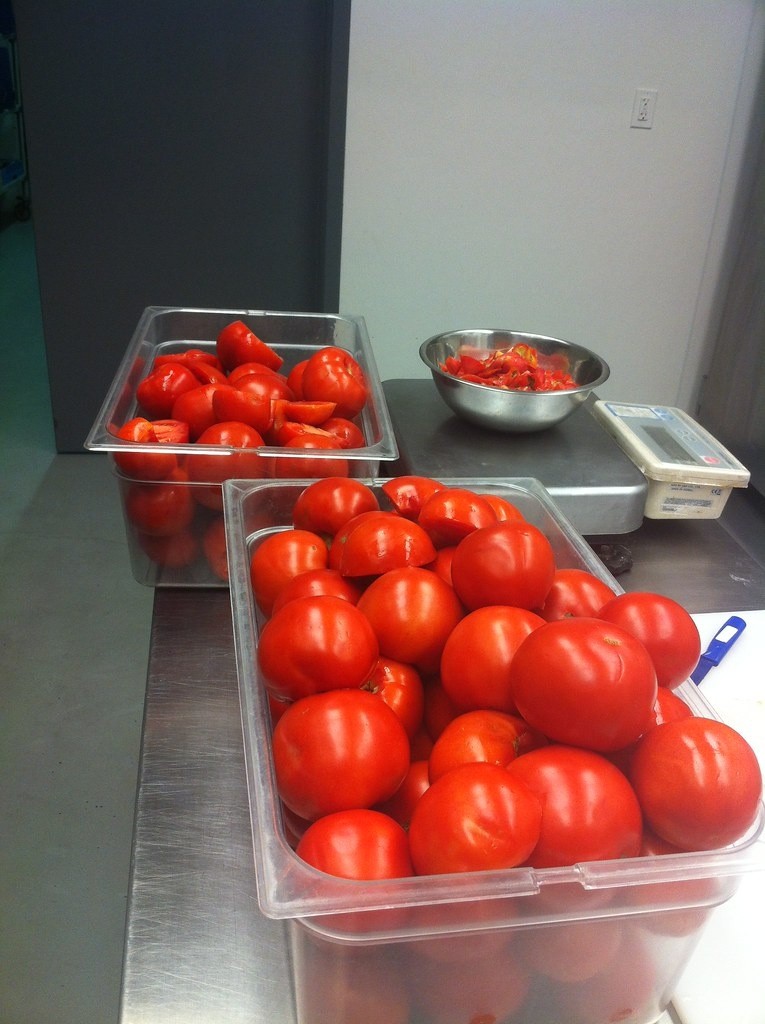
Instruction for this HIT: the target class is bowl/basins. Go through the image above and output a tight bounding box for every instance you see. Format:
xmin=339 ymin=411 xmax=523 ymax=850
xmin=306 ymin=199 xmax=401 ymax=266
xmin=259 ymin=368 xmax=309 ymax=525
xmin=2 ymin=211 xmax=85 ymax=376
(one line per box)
xmin=419 ymin=328 xmax=611 ymax=431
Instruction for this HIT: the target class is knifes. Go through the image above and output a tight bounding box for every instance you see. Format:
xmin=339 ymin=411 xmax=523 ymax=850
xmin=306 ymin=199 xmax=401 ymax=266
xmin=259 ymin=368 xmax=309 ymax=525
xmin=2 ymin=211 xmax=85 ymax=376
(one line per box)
xmin=693 ymin=616 xmax=746 ymax=685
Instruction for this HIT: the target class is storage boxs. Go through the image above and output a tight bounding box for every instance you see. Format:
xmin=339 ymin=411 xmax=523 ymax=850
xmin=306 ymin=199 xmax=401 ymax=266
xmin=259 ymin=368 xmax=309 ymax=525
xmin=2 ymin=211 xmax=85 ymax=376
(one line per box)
xmin=84 ymin=305 xmax=400 ymax=587
xmin=223 ymin=477 xmax=765 ymax=1024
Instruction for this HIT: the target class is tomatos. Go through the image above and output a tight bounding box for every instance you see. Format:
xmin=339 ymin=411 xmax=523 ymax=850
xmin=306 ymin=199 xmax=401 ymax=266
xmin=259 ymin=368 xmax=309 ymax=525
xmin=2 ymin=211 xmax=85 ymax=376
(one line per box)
xmin=248 ymin=477 xmax=763 ymax=1024
xmin=116 ymin=320 xmax=368 ymax=578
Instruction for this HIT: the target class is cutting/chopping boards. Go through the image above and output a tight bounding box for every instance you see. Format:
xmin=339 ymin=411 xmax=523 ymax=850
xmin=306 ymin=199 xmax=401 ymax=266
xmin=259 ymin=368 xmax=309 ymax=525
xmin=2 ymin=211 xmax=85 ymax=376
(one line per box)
xmin=657 ymin=609 xmax=765 ymax=1024
